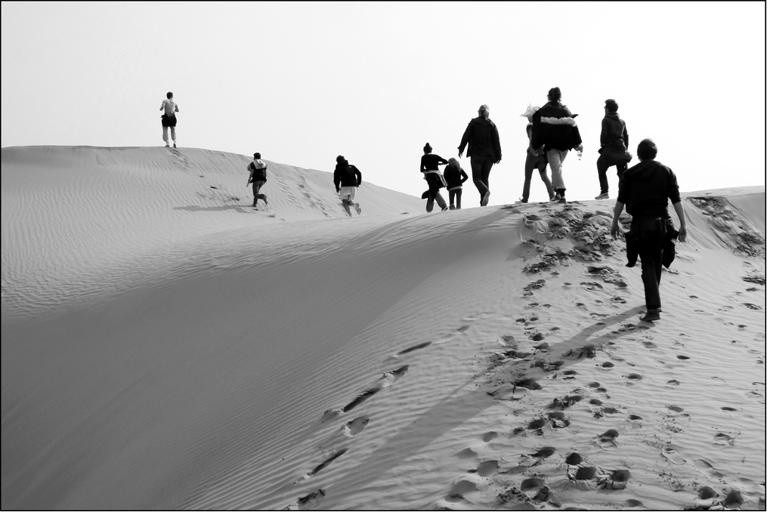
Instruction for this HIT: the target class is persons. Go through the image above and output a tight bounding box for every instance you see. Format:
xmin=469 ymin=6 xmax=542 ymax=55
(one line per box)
xmin=246 ymin=152 xmax=267 ymax=208
xmin=457 ymin=104 xmax=501 ymax=207
xmin=532 ymin=87 xmax=584 ymax=202
xmin=420 ymin=143 xmax=449 ymax=211
xmin=595 ymin=98 xmax=632 ymax=198
xmin=160 ymin=91 xmax=179 ymax=148
xmin=333 ymin=155 xmax=362 ymax=219
xmin=444 ymin=158 xmax=468 ymax=209
xmin=516 ymin=104 xmax=555 ymax=203
xmin=612 ymin=139 xmax=687 ymax=321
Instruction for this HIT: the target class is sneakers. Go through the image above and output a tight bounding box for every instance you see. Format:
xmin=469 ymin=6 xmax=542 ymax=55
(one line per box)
xmin=640 ymin=311 xmax=661 ymax=320
xmin=595 ymin=193 xmax=609 ymax=200
xmin=441 ymin=206 xmax=448 ymax=211
xmin=354 ymin=202 xmax=361 ymax=215
xmin=480 ymin=190 xmax=490 ymax=206
